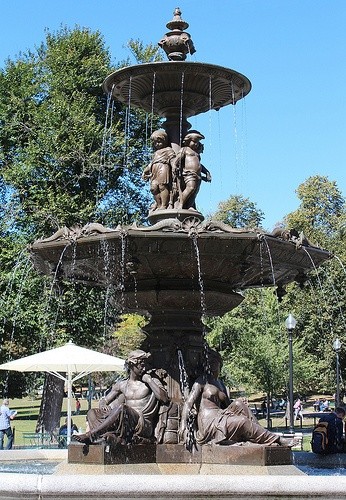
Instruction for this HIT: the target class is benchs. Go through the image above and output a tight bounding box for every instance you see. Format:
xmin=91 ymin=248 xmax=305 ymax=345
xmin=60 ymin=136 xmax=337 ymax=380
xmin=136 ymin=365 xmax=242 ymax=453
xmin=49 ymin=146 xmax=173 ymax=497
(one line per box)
xmin=21 ymin=431 xmax=67 ymax=447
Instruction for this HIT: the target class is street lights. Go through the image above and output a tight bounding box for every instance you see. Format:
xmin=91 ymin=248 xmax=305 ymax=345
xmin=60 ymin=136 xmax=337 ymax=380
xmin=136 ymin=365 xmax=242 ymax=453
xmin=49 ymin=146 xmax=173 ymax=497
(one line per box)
xmin=332 ymin=337 xmax=344 ymax=410
xmin=284 ymin=312 xmax=298 ymax=434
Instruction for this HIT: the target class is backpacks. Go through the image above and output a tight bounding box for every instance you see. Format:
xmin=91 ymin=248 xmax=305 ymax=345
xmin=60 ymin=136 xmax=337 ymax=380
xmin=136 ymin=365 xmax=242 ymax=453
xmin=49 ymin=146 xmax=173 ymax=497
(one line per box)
xmin=311 ymin=416 xmax=338 ymax=455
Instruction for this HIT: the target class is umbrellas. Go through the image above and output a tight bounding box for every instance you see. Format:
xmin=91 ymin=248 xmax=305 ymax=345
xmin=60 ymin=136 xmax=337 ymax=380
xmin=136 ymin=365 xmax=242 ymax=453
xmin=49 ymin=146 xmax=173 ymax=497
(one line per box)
xmin=0 ymin=340 xmax=125 ymax=445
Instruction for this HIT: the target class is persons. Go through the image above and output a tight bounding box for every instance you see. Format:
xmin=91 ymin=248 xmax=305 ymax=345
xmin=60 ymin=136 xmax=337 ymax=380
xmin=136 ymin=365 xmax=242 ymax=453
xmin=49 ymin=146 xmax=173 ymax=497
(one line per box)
xmin=293 ymin=399 xmax=303 ymax=423
xmin=261 ymin=398 xmax=286 ymax=409
xmin=0 ymin=399 xmax=17 ymax=450
xmin=73 ymin=349 xmax=172 ymax=446
xmin=176 ymin=349 xmax=300 ymax=447
xmin=313 ymin=398 xmax=330 ymax=413
xmin=59 ymin=417 xmax=79 ymax=448
xmin=173 ymin=130 xmax=211 ymax=212
xmin=319 ymin=407 xmax=346 ymax=453
xmin=75 ymin=399 xmax=81 ymax=414
xmin=142 ymin=128 xmax=179 ymax=211
